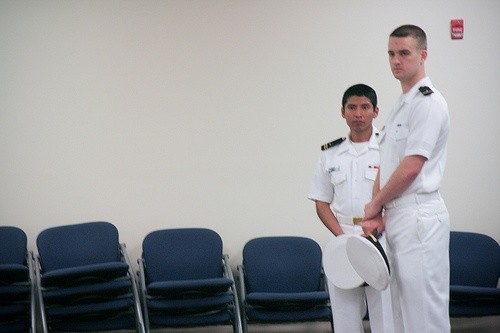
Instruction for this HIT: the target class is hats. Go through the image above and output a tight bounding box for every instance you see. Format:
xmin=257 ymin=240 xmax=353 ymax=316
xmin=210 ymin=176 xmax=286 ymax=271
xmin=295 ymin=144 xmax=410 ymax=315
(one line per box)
xmin=345 ymin=233 xmax=394 ymax=292
xmin=323 ymin=234 xmax=369 ymax=290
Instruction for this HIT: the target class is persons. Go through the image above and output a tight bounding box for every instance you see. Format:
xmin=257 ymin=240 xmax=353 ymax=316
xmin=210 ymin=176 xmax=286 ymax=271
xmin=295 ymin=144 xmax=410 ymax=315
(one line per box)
xmin=358 ymin=24 xmax=451 ymax=333
xmin=308 ymin=84 xmax=394 ymax=333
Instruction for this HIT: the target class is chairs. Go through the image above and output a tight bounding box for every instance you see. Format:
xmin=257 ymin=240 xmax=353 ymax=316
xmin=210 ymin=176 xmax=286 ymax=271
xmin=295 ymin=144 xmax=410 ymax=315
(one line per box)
xmin=0 ymin=222 xmax=500 ymax=333
